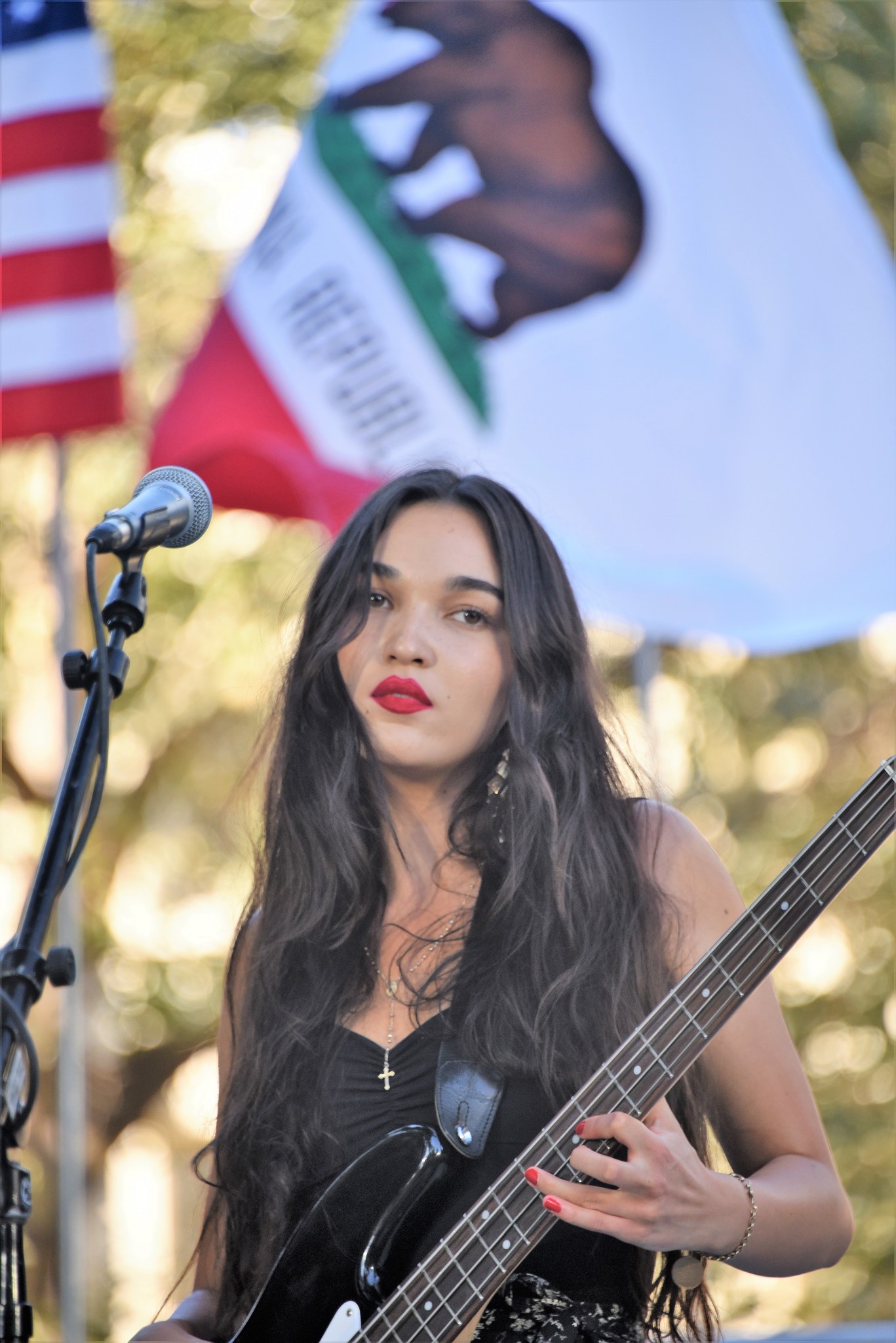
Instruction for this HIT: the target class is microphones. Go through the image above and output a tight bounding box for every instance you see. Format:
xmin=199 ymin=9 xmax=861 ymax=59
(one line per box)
xmin=85 ymin=465 xmax=213 ymax=554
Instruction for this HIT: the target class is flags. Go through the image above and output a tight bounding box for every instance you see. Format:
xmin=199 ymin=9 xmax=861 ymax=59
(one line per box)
xmin=148 ymin=1 xmax=896 ymax=656
xmin=0 ymin=1 xmax=131 ymax=442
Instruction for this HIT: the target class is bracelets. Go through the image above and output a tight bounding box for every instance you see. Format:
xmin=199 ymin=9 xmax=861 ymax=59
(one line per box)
xmin=696 ymin=1173 xmax=758 ymax=1261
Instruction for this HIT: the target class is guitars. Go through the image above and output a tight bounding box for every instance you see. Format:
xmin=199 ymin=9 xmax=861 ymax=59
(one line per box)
xmin=220 ymin=755 xmax=896 ymax=1343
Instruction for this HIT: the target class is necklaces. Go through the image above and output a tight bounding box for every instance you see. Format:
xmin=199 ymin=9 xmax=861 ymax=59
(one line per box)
xmin=363 ymin=872 xmax=479 ymax=1091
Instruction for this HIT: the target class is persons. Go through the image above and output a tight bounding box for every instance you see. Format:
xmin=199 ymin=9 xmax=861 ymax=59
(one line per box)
xmin=126 ymin=466 xmax=854 ymax=1343
